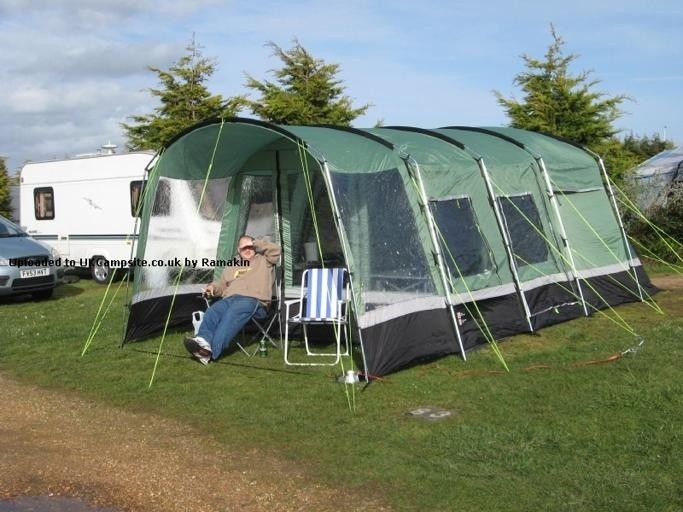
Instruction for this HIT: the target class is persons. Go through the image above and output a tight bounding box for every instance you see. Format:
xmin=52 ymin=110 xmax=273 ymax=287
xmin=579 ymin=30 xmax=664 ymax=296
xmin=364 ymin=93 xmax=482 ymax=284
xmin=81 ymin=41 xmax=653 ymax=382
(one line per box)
xmin=182 ymin=234 xmax=281 ymax=365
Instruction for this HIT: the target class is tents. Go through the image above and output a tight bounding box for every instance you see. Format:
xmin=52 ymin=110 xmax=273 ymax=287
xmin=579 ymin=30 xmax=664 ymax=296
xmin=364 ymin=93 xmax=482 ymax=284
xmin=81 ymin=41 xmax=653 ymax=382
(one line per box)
xmin=117 ymin=117 xmax=666 ymax=382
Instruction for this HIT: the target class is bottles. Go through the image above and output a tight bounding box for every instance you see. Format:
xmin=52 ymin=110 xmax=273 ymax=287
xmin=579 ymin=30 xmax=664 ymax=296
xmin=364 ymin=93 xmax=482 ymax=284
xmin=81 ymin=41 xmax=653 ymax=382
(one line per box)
xmin=260 ymin=340 xmax=267 ymax=357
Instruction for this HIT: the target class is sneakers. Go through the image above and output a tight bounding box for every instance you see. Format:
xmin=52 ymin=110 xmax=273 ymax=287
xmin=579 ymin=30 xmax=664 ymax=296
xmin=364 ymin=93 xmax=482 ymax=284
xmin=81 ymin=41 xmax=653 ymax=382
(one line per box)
xmin=182 ymin=335 xmax=213 ymax=365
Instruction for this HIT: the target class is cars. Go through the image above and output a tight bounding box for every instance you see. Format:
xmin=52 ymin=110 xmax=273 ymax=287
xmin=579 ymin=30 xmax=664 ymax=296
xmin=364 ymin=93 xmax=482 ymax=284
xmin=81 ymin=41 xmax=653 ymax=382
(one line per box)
xmin=0 ymin=214 xmax=57 ymax=302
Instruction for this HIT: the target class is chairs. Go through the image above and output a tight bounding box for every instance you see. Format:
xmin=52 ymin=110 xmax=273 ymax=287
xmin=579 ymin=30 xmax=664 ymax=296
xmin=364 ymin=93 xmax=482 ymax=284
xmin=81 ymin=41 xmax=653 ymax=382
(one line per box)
xmin=196 ymin=276 xmax=284 ymax=358
xmin=283 ymin=268 xmax=352 ymax=366
